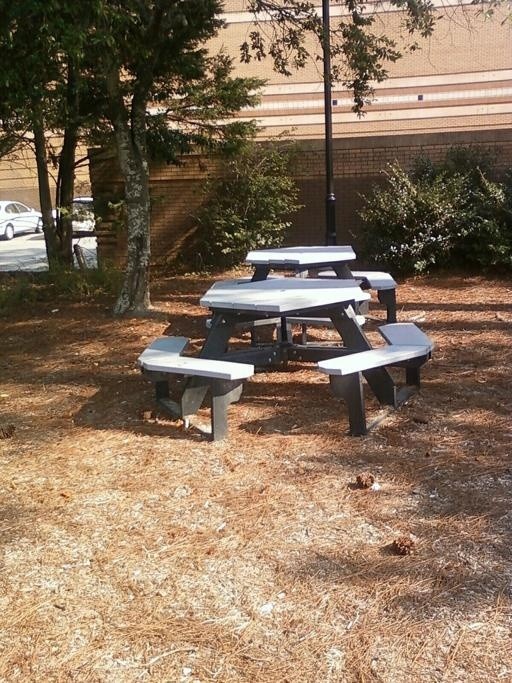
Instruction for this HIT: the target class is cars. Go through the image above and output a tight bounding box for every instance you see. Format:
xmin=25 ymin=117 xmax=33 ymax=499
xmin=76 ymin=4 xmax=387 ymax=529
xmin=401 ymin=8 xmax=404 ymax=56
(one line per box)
xmin=1 ymin=201 xmax=44 ymax=240
xmin=50 ymin=196 xmax=96 ymax=236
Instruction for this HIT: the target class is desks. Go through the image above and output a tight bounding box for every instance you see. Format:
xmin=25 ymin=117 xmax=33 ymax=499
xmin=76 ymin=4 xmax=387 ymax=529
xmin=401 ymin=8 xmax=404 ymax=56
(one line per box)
xmin=180 ymin=278 xmax=397 ymax=423
xmin=247 ymin=243 xmax=370 ymax=316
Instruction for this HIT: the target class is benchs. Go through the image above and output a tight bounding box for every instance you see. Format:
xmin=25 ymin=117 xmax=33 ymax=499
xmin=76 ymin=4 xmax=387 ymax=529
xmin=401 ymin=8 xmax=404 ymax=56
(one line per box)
xmin=314 ymin=268 xmax=397 ymax=323
xmin=318 ymin=323 xmax=437 ymax=436
xmin=142 ymin=337 xmax=252 ymax=441
xmin=211 ymin=275 xmax=284 ymax=287
